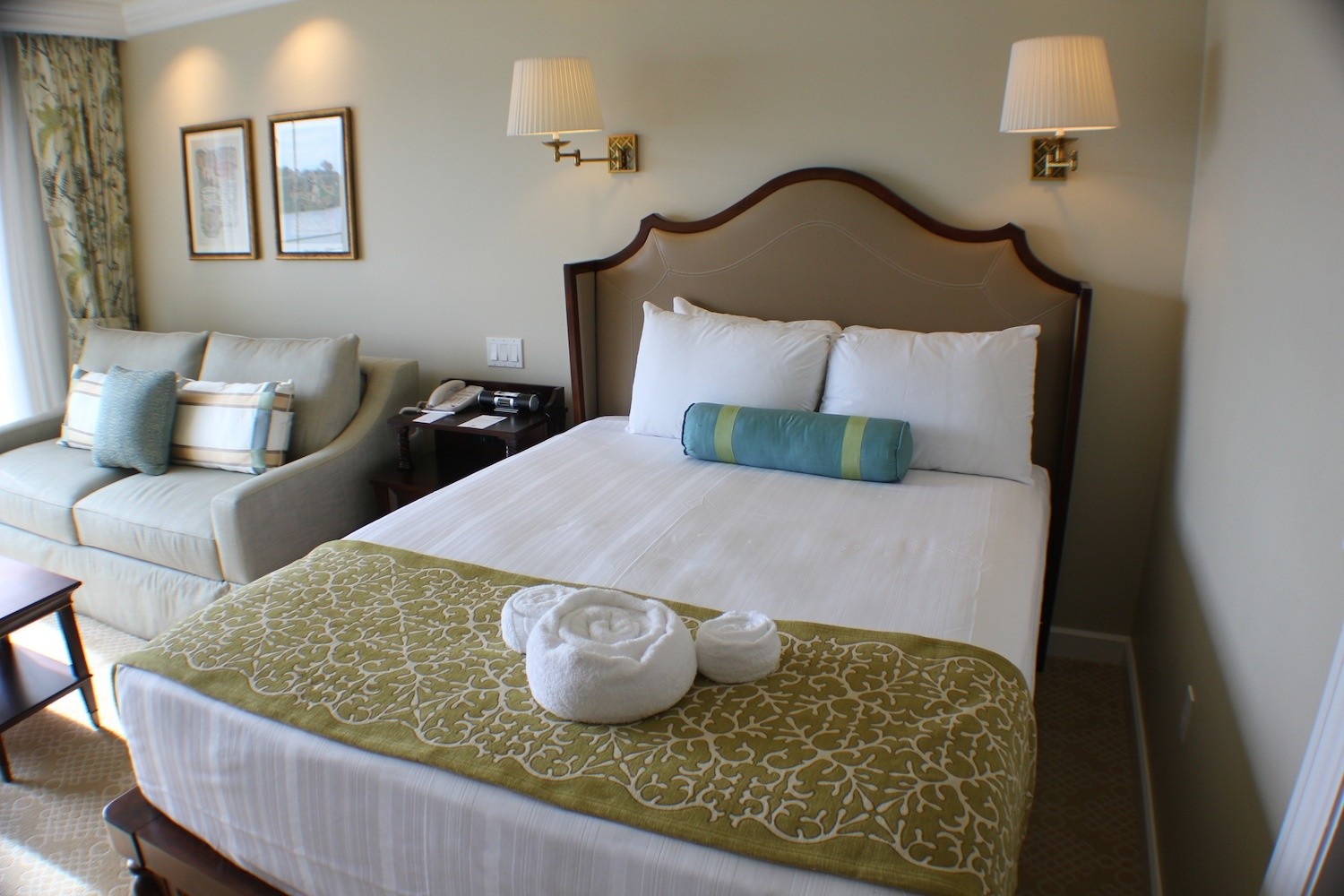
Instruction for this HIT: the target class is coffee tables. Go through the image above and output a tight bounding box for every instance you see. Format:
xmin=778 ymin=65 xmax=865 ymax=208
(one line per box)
xmin=0 ymin=554 xmax=98 ymax=786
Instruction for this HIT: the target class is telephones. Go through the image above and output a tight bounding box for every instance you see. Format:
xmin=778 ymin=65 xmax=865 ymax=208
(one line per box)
xmin=424 ymin=379 xmax=485 ymax=413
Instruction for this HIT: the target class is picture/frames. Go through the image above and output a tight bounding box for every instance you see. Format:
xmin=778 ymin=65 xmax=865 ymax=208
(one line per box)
xmin=180 ymin=117 xmax=257 ymax=260
xmin=267 ymin=106 xmax=356 ymax=260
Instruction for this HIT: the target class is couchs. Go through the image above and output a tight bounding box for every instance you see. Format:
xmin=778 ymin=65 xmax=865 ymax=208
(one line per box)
xmin=0 ymin=354 xmax=420 ymax=640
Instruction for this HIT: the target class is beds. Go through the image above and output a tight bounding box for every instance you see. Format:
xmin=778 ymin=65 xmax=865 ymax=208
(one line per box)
xmin=99 ymin=166 xmax=1095 ymax=896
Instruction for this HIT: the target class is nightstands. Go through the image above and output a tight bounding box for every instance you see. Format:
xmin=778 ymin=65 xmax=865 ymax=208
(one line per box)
xmin=367 ymin=379 xmax=570 ymax=516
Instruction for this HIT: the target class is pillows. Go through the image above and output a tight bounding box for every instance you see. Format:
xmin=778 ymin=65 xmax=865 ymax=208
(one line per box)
xmin=264 ymin=378 xmax=293 ymax=473
xmin=670 ymin=294 xmax=842 ymax=412
xmin=55 ymin=361 xmax=107 ymax=452
xmin=165 ymin=369 xmax=280 ymax=477
xmin=681 ymin=402 xmax=913 ymax=485
xmin=92 ymin=362 xmax=178 ymax=477
xmin=819 ymin=323 xmax=1045 ymax=488
xmin=626 ymin=300 xmax=832 ymax=440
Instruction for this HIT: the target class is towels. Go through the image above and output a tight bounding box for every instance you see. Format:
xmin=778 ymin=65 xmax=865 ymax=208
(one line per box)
xmin=691 ymin=609 xmax=781 ymax=680
xmin=500 ymin=585 xmax=579 ymax=653
xmin=525 ymin=585 xmax=696 ymax=723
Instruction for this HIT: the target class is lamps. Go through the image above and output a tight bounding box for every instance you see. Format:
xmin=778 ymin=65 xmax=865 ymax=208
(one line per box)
xmin=507 ymin=58 xmax=639 ymax=173
xmin=999 ymin=33 xmax=1118 ymax=182
